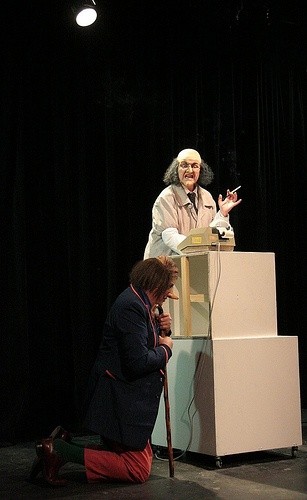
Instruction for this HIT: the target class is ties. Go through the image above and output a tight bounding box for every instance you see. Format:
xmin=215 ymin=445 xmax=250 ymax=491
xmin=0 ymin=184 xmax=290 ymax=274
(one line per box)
xmin=187 ymin=192 xmax=196 ymax=209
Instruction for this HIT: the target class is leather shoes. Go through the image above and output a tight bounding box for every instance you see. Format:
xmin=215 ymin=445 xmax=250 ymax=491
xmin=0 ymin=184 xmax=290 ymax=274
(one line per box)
xmin=30 ymin=424 xmax=69 ymax=486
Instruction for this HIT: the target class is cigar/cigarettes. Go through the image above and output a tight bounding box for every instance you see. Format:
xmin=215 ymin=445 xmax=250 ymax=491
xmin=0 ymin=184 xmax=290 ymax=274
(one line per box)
xmin=226 ymin=185 xmax=242 ymax=197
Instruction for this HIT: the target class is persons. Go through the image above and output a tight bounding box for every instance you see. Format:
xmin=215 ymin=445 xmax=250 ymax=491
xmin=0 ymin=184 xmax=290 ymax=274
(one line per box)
xmin=31 ymin=256 xmax=179 ymax=486
xmin=142 ymin=148 xmax=242 ymax=261
